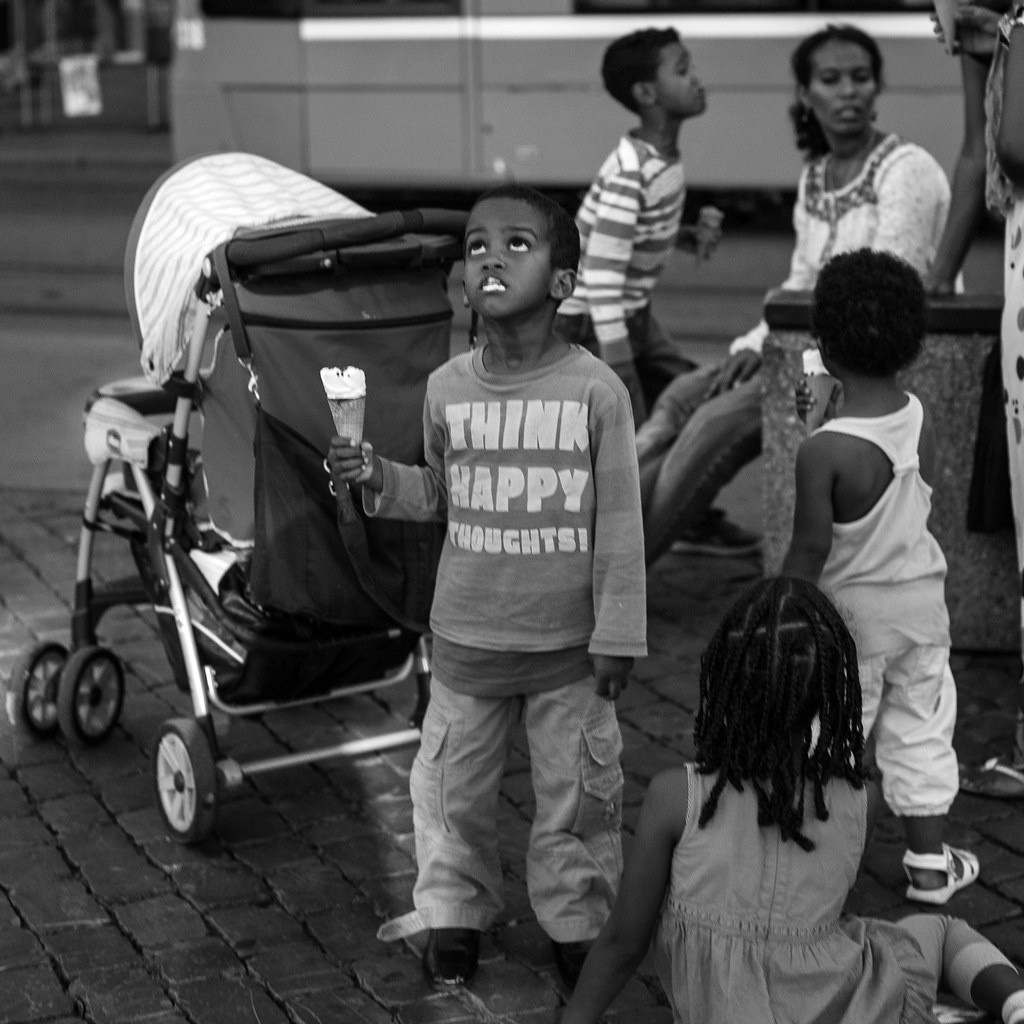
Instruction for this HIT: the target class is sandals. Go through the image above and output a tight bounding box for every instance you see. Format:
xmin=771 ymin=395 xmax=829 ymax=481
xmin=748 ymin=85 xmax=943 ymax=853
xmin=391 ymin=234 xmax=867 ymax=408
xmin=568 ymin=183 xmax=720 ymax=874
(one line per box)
xmin=902 ymin=841 xmax=980 ymax=905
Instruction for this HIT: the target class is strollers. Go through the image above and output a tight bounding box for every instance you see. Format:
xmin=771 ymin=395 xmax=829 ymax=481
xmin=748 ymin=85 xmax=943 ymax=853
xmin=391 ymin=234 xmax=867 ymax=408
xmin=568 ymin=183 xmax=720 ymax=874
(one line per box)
xmin=8 ymin=148 xmax=478 ymax=848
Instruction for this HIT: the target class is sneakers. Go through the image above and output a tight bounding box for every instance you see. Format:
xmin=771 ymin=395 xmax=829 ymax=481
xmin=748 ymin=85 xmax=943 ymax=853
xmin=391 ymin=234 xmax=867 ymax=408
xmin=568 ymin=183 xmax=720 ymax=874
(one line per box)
xmin=669 ymin=508 xmax=766 ymax=556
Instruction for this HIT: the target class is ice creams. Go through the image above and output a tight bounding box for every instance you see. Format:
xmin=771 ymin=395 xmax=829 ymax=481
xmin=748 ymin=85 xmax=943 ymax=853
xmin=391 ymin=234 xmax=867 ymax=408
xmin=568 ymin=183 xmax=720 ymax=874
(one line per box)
xmin=934 ymin=0 xmax=959 ymax=56
xmin=319 ymin=366 xmax=366 ymax=447
xmin=802 ymin=347 xmax=836 ymax=434
xmin=696 ymin=206 xmax=725 ymax=258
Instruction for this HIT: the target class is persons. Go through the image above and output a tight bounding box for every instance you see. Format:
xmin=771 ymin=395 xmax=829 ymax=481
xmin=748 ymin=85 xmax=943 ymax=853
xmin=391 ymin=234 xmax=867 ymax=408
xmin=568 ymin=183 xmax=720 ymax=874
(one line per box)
xmin=931 ymin=0 xmax=1024 ymax=799
xmin=328 ymin=180 xmax=651 ymax=989
xmin=559 ymin=575 xmax=1023 ymax=1024
xmin=640 ymin=24 xmax=951 ymax=568
xmin=555 ymin=27 xmax=769 ymax=556
xmin=919 ymin=54 xmax=992 ymax=292
xmin=780 ymin=248 xmax=982 ymax=905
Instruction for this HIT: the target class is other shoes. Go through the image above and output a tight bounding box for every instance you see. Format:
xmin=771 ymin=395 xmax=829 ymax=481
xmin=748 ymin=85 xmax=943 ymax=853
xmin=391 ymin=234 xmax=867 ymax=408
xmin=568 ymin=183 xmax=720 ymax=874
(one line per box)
xmin=554 ymin=951 xmax=584 ymax=986
xmin=1001 ymin=990 xmax=1024 ymax=1024
xmin=420 ymin=928 xmax=482 ymax=994
xmin=958 ymin=733 xmax=1024 ymax=798
